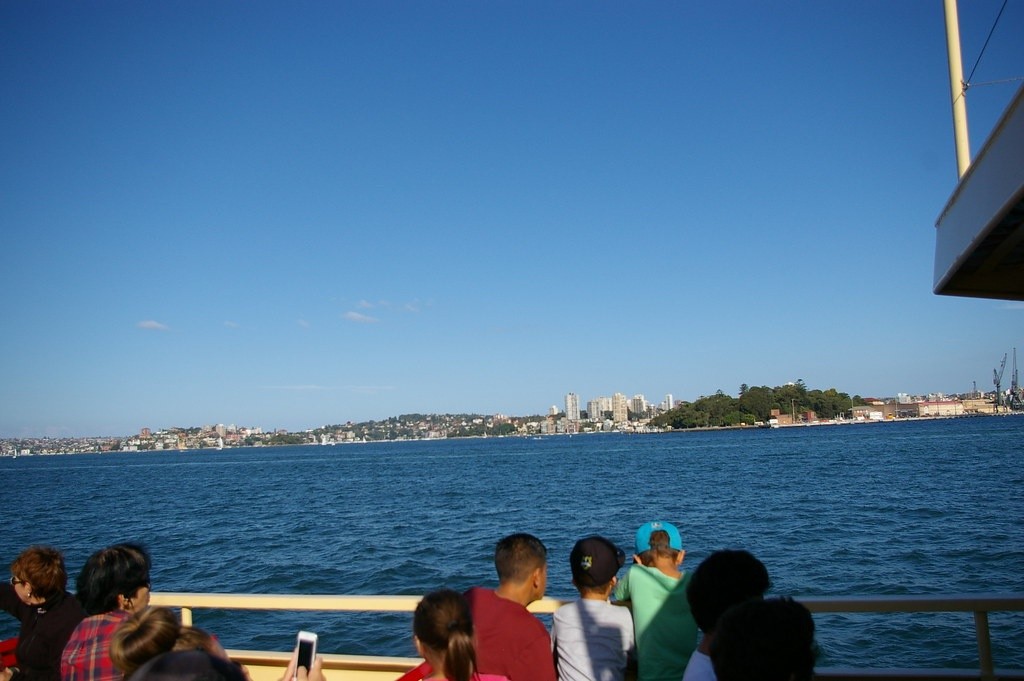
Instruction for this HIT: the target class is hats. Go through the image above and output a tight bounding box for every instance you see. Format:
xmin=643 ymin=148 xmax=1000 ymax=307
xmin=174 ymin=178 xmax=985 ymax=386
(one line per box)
xmin=633 ymin=520 xmax=682 ymax=564
xmin=570 ymin=536 xmax=626 ymax=587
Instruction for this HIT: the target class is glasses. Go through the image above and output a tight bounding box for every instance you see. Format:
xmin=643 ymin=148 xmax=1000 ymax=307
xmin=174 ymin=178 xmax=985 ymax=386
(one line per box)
xmin=126 ymin=575 xmax=151 ymax=600
xmin=10 ymin=576 xmax=28 ymax=586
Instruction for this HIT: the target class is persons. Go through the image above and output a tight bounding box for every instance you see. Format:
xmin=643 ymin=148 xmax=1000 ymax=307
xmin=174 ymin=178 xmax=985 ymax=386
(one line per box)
xmin=0 ymin=543 xmax=82 ymax=681
xmin=60 ymin=542 xmax=152 ymax=681
xmin=681 ymin=548 xmax=773 ymax=680
xmin=614 ymin=520 xmax=699 ymax=681
xmin=108 ymin=607 xmax=326 ymax=681
xmin=461 ymin=533 xmax=557 ymax=681
xmin=411 ymin=589 xmax=509 ymax=681
xmin=550 ymin=535 xmax=634 ymax=681
xmin=709 ymin=594 xmax=817 ymax=681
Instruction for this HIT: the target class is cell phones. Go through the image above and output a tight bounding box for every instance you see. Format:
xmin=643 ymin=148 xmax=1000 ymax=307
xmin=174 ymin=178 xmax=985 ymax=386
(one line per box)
xmin=293 ymin=630 xmax=318 ymax=681
